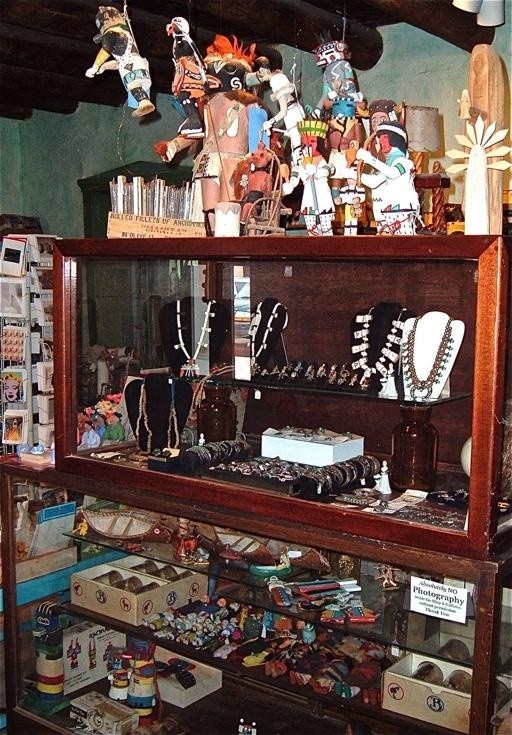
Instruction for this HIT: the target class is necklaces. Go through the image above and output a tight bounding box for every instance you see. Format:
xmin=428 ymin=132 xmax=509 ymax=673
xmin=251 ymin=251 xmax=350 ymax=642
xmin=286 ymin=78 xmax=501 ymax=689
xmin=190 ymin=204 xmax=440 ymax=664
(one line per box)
xmin=351 ymin=305 xmax=409 ymax=391
xmin=400 ymin=313 xmax=459 ymax=402
xmin=170 ymin=293 xmax=221 ymax=375
xmin=134 ymin=373 xmax=181 ymax=456
xmin=247 ymin=300 xmax=284 ymax=376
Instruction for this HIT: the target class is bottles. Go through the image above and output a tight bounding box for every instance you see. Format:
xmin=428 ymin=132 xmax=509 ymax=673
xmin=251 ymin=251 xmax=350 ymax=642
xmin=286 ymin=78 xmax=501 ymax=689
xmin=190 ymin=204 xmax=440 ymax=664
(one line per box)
xmin=197 ymin=386 xmax=237 ymax=443
xmin=388 ymin=401 xmax=441 ymax=492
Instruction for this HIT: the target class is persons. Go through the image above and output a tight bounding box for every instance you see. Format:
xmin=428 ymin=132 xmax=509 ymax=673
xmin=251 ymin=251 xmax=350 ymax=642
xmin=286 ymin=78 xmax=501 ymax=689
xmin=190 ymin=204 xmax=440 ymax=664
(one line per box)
xmin=3 ymin=372 xmax=25 ymax=403
xmin=153 ymin=16 xmax=427 ymax=239
xmin=49 ymin=409 xmax=126 ymax=466
xmin=142 ymin=593 xmax=388 ymax=705
xmin=85 ymin=5 xmax=157 ymax=119
xmin=4 ymin=417 xmax=21 ymax=441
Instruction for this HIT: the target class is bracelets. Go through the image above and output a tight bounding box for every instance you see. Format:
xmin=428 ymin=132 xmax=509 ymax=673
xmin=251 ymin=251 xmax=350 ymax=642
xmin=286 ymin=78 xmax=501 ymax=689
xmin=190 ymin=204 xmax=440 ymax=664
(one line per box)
xmin=185 ymin=360 xmax=376 ymax=499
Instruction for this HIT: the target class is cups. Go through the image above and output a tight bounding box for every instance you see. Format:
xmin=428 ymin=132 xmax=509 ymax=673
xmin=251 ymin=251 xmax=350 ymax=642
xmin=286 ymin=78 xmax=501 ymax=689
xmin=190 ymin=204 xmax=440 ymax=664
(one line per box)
xmin=431 ymin=638 xmax=498 ymax=666
xmin=411 ymin=654 xmax=512 ymax=704
